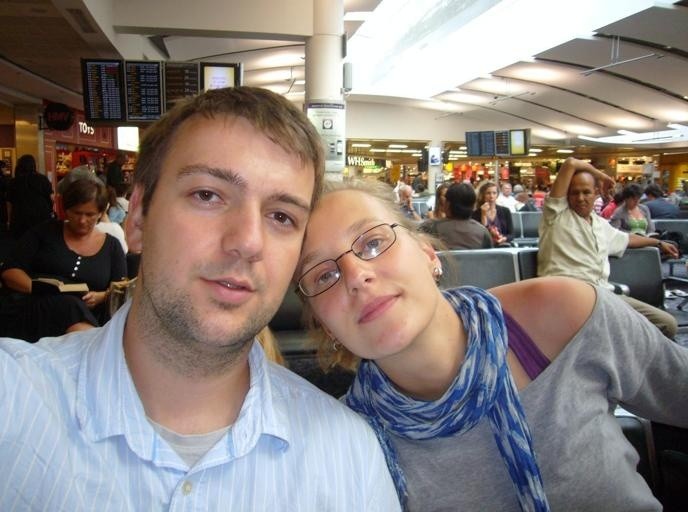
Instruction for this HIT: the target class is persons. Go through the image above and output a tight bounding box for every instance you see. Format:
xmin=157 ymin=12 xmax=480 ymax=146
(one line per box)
xmin=294 ymin=177 xmax=687 ymax=511
xmin=595 ymin=173 xmax=688 ymax=235
xmin=392 ymin=163 xmax=535 ymax=251
xmin=5 ymin=155 xmax=55 ymax=231
xmin=536 ymin=156 xmax=680 ymax=342
xmin=0 ymin=165 xmax=126 ymax=335
xmin=534 ymin=160 xmax=557 ymax=206
xmin=2 ymin=87 xmax=406 ymax=512
xmin=55 ymin=153 xmax=94 ymax=183
xmin=100 ymin=151 xmax=138 ymax=235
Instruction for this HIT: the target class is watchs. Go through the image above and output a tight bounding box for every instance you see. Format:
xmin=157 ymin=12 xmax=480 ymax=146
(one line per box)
xmin=657 ymin=240 xmax=664 ymax=248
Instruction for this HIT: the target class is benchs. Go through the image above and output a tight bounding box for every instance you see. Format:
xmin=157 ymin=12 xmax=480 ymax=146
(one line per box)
xmin=652 ymin=219 xmax=688 ymax=308
xmin=267 ymin=282 xmax=358 ymax=360
xmin=512 ymin=211 xmax=541 ymax=247
xmin=437 ymin=249 xmax=688 ymax=333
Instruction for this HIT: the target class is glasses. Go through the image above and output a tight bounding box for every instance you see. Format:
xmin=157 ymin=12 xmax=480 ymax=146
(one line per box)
xmin=293 ymin=223 xmax=410 ymax=303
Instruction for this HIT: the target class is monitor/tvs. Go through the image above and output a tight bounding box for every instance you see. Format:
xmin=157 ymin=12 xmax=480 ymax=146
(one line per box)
xmin=81 ymin=61 xmax=245 ymax=124
xmin=464 ymin=128 xmax=532 ymax=158
